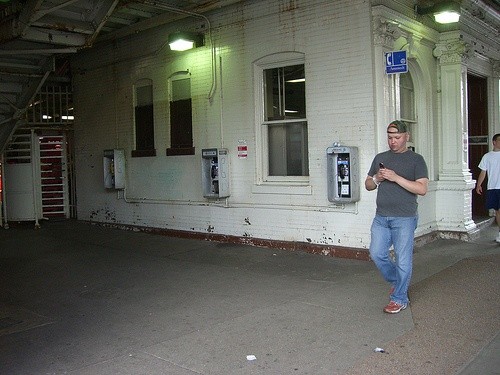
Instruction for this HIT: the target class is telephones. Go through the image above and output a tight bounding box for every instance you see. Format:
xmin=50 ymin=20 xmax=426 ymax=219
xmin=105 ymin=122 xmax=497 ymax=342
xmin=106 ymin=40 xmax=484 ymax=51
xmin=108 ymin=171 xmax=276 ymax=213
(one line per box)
xmin=337 ymin=156 xmax=349 ymax=183
xmin=110 ymin=163 xmax=114 ymax=173
xmin=209 ymin=162 xmax=218 ymax=181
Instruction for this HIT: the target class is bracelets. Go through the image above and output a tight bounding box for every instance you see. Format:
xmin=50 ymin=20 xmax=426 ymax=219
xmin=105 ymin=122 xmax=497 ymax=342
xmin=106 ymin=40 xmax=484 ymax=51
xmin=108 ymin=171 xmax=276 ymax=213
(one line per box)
xmin=373 ymin=175 xmax=381 ymax=188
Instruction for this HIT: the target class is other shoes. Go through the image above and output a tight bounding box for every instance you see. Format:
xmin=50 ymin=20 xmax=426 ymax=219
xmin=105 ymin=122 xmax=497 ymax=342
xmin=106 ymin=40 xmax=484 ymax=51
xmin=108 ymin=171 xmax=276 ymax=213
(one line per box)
xmin=496 ymin=232 xmax=500 ymax=242
xmin=383 ymin=302 xmax=407 ymax=313
xmin=388 ymin=288 xmax=394 ymax=295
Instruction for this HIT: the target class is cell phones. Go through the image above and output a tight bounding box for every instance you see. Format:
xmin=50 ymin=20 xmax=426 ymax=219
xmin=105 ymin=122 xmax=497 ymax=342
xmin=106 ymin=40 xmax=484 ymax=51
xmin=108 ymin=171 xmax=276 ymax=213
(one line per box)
xmin=380 ymin=162 xmax=384 ymax=169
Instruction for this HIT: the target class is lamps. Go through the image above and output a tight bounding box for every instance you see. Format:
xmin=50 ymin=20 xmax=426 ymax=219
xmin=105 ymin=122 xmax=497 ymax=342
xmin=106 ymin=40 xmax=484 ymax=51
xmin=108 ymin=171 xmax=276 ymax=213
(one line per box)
xmin=414 ymin=0 xmax=462 ymax=24
xmin=168 ymin=31 xmax=205 ymax=52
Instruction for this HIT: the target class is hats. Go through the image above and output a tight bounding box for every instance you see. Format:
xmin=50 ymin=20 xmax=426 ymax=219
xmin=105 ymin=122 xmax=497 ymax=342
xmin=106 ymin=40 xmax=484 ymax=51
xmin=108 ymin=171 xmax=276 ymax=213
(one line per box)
xmin=387 ymin=120 xmax=408 ymax=134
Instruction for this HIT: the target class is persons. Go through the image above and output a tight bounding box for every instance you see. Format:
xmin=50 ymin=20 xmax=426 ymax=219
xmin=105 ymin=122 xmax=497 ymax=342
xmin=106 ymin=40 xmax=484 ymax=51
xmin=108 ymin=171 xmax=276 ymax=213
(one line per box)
xmin=476 ymin=134 xmax=500 ymax=243
xmin=365 ymin=120 xmax=430 ymax=314
